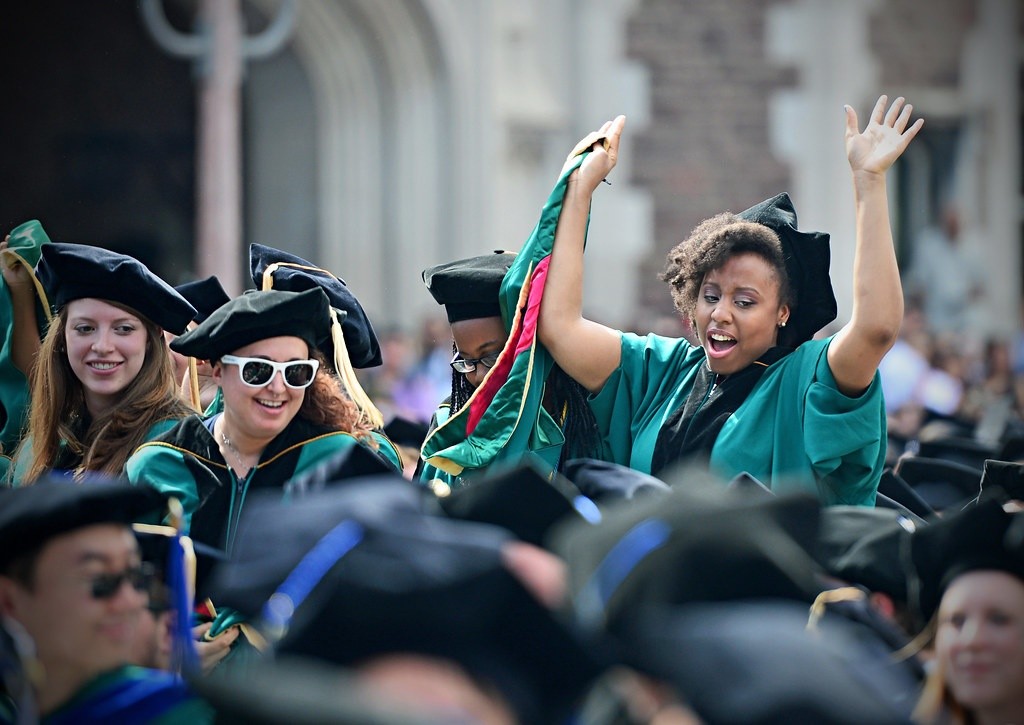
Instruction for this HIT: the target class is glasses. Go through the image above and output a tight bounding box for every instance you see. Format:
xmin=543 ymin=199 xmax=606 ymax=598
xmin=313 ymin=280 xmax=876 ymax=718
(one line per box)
xmin=449 ymin=351 xmax=500 ymax=374
xmin=221 ymin=354 xmax=321 ymax=389
xmin=30 ymin=563 xmax=153 ymax=598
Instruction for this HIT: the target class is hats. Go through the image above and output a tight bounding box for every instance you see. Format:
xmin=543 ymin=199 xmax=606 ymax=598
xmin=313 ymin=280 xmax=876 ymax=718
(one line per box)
xmin=250 ymin=242 xmax=384 ymax=369
xmin=33 ymin=242 xmax=203 ymax=414
xmin=4 ymin=420 xmax=1023 ymax=725
xmin=169 ymin=286 xmax=385 ymax=430
xmin=737 ymin=192 xmax=837 ymax=365
xmin=422 ymin=250 xmax=517 ymax=324
xmin=173 ymin=276 xmax=232 ymax=325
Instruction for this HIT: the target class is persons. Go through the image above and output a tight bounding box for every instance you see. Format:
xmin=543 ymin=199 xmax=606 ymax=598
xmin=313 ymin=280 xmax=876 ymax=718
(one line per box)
xmin=0 ymin=94 xmax=1024 ymax=725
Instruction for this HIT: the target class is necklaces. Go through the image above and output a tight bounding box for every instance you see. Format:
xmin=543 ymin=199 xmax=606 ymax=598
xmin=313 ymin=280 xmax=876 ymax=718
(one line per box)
xmin=220 ymin=430 xmax=251 ymax=473
